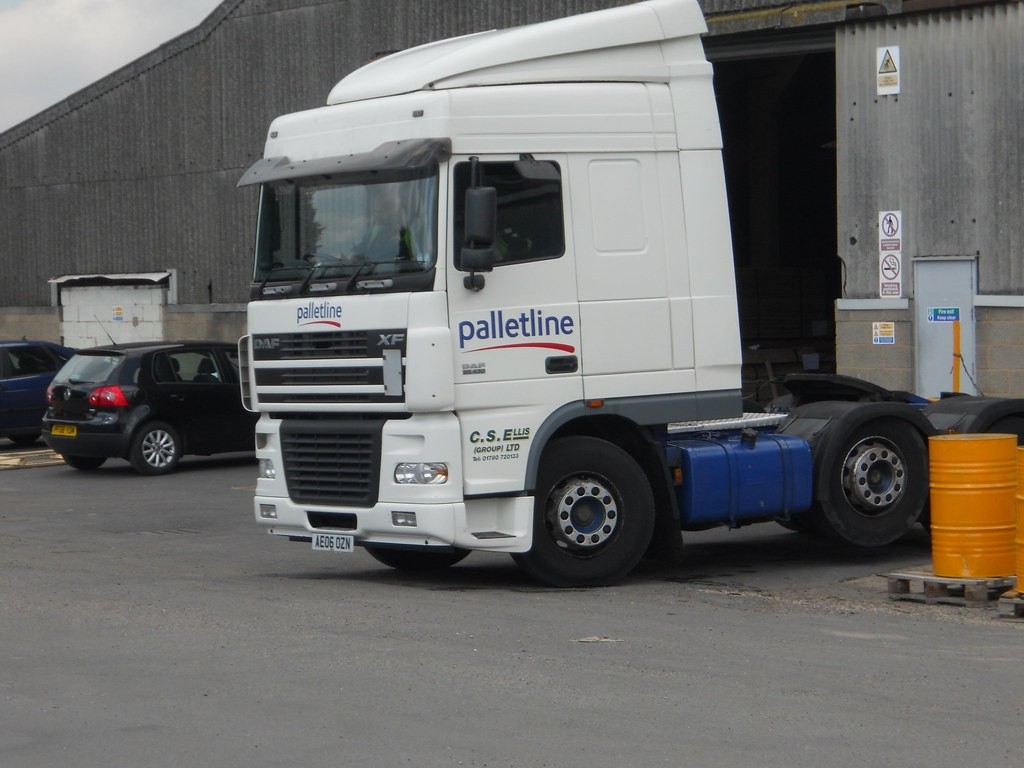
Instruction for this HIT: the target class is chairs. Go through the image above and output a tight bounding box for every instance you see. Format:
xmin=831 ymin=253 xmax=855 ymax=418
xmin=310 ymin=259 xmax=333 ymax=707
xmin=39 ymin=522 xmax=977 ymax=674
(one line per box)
xmin=16 ymin=359 xmax=37 ymax=376
xmin=494 ymin=200 xmax=534 ymax=263
xmin=193 ymin=359 xmax=219 ymax=383
xmin=170 ymin=356 xmax=182 ymax=381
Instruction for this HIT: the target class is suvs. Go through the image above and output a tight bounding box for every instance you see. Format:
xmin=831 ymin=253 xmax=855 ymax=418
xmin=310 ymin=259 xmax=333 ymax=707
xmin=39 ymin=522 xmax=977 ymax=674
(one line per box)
xmin=40 ymin=340 xmax=261 ymax=476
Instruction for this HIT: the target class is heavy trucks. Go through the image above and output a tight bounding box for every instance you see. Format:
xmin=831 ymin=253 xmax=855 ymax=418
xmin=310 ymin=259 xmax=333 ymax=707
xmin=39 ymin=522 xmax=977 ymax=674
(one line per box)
xmin=235 ymin=0 xmax=1024 ymax=589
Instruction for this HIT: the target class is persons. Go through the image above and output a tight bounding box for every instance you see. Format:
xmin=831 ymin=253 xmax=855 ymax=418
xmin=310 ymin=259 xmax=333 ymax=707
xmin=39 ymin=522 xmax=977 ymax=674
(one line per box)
xmin=313 ymin=192 xmax=413 ymax=263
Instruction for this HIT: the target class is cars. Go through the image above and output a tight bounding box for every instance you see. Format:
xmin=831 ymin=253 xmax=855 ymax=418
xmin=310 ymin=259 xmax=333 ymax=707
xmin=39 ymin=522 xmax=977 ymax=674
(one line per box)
xmin=0 ymin=335 xmax=80 ymax=444
